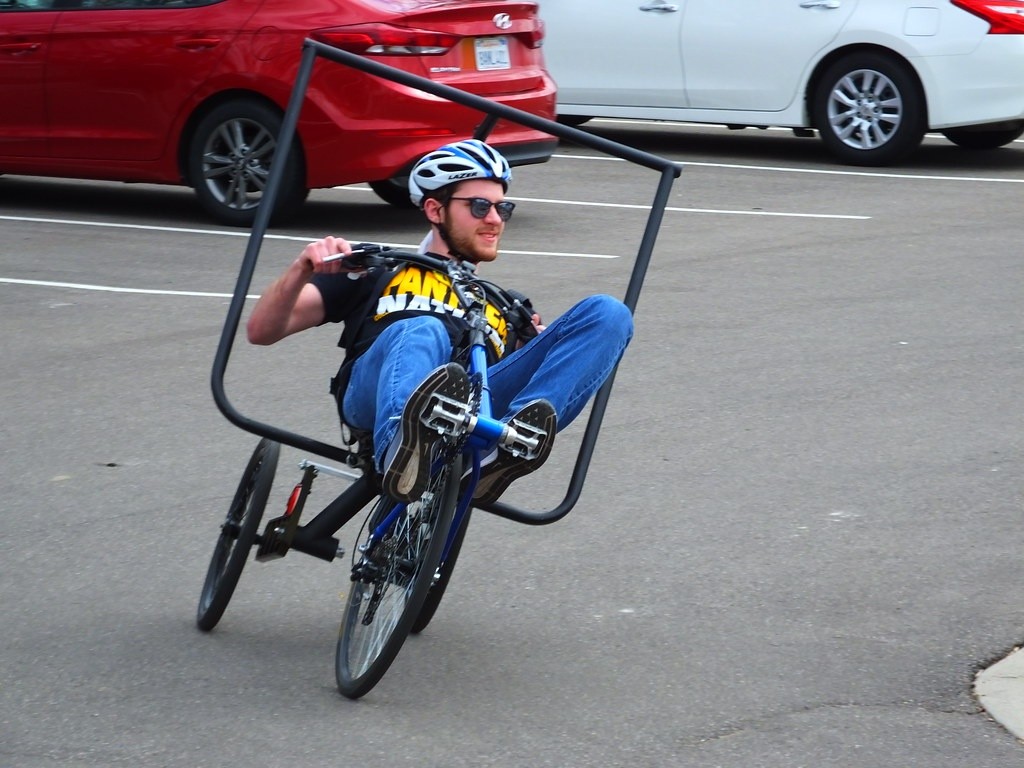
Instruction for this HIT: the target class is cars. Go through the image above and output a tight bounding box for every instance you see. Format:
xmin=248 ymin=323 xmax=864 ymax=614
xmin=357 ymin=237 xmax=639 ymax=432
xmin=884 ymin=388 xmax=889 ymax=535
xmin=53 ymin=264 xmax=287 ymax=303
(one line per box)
xmin=536 ymin=0 xmax=1024 ymax=167
xmin=0 ymin=0 xmax=560 ymax=228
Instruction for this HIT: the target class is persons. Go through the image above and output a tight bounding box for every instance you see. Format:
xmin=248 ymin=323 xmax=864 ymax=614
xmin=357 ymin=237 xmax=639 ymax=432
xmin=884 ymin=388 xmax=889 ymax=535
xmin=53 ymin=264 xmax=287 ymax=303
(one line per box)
xmin=245 ymin=141 xmax=636 ymax=505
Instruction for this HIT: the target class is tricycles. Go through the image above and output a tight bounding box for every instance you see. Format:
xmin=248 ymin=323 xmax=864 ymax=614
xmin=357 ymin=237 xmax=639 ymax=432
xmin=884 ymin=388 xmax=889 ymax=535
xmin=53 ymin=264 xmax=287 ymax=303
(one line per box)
xmin=198 ymin=38 xmax=683 ymax=696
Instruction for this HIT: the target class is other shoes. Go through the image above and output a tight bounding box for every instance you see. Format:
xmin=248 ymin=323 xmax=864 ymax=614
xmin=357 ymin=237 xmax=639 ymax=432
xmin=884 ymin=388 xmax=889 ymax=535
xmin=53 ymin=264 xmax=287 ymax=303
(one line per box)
xmin=382 ymin=361 xmax=469 ymax=501
xmin=457 ymin=398 xmax=558 ymax=510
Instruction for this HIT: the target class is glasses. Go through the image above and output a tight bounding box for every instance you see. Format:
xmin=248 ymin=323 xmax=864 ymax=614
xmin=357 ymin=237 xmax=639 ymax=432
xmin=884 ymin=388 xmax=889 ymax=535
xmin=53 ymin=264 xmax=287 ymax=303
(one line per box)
xmin=444 ymin=195 xmax=518 ymax=222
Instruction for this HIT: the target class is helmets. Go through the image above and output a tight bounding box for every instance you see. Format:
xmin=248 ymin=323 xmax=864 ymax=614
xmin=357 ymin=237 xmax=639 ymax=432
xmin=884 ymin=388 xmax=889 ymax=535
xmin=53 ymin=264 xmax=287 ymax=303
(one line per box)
xmin=409 ymin=140 xmax=512 ymax=206
